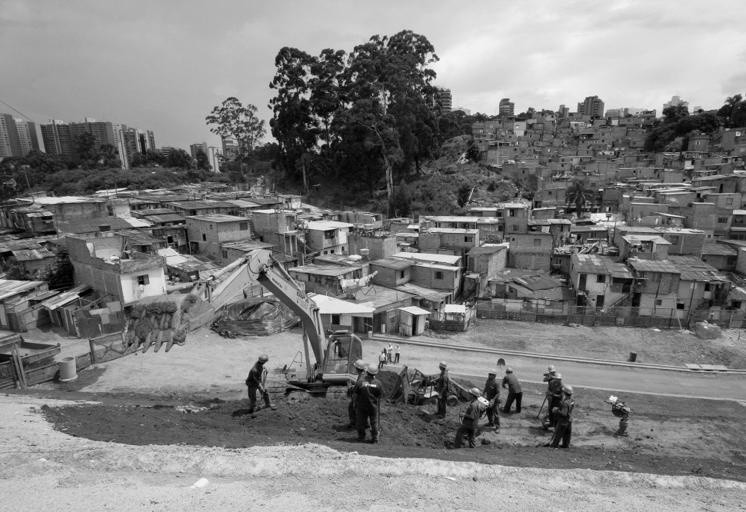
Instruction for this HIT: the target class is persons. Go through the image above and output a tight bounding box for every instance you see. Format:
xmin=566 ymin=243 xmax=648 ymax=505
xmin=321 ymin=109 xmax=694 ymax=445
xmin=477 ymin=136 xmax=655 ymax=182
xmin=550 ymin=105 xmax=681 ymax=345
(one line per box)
xmin=246 ymin=354 xmax=276 ymax=412
xmin=338 ymin=342 xmax=349 ymax=358
xmin=454 ymin=387 xmax=480 ymax=449
xmin=543 ymin=365 xmax=563 ymax=427
xmin=501 ymin=368 xmax=523 ymax=413
xmin=346 ymin=364 xmax=384 ymax=444
xmin=482 ymin=372 xmax=501 ymax=434
xmin=430 ymin=362 xmax=449 ymax=419
xmin=346 ymin=359 xmax=370 ymax=429
xmin=549 ymin=385 xmax=575 ymax=449
xmin=377 ymin=344 xmax=401 ymax=370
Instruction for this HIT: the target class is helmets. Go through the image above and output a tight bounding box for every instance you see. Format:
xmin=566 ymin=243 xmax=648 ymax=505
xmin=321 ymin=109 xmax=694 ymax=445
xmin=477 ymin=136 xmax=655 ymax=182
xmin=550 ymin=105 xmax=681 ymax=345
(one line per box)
xmin=506 ymin=367 xmax=513 ymax=372
xmin=439 ymin=361 xmax=447 ymax=368
xmin=488 ymin=369 xmax=497 ymax=375
xmin=259 ymin=355 xmax=268 ymax=362
xmin=551 ymin=373 xmax=562 ymax=379
xmin=354 ymin=360 xmax=366 ymax=369
xmin=548 ymin=365 xmax=556 ymax=372
xmin=562 ymin=385 xmax=573 ymax=394
xmin=469 ymin=388 xmax=480 ymax=397
xmin=367 ymin=364 xmax=378 ymax=374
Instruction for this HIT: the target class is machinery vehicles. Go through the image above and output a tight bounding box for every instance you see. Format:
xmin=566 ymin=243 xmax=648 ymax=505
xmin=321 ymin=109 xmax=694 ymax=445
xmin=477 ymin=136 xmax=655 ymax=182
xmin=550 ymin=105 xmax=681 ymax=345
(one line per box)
xmin=390 ymin=364 xmax=472 ymax=407
xmin=120 ymin=248 xmax=364 ymax=403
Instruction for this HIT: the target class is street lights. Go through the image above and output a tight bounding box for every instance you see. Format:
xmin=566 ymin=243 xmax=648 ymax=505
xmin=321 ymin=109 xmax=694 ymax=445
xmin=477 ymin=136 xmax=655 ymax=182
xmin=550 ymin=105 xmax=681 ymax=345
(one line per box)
xmin=21 ymin=164 xmax=35 ymax=204
